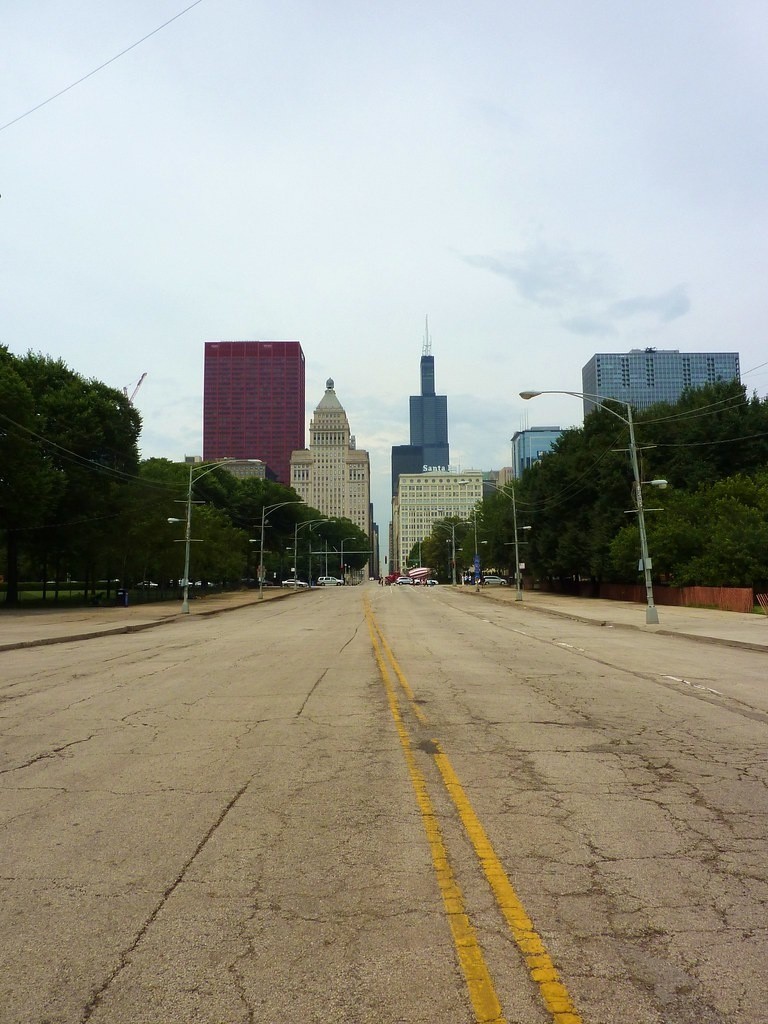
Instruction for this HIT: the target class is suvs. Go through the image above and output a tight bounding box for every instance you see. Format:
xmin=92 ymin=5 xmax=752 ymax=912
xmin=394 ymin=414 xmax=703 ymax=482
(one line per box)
xmin=316 ymin=577 xmax=345 ymax=586
xmin=396 ymin=576 xmax=413 ymax=586
xmin=482 ymin=575 xmax=507 ymax=586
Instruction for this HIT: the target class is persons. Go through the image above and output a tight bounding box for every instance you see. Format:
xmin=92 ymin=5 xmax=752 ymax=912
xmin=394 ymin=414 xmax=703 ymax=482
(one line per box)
xmin=461 ymin=574 xmax=486 ymax=588
xmin=379 ymin=577 xmax=391 ymax=587
xmin=412 ymin=577 xmax=434 ymax=587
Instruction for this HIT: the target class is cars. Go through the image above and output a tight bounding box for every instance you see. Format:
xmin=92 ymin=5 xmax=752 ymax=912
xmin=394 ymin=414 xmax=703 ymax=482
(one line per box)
xmin=410 ymin=578 xmax=420 ymax=585
xmin=170 ymin=578 xmax=211 ymax=586
xmin=282 ymin=579 xmax=308 ymax=587
xmin=137 ymin=582 xmax=158 ymax=587
xmin=427 ymin=579 xmax=439 ymax=585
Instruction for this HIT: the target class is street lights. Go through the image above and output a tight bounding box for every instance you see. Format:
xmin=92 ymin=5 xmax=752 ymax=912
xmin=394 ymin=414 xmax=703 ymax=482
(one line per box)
xmin=431 ymin=520 xmax=472 ymax=587
xmin=458 ymin=481 xmax=532 ymax=600
xmin=285 ymin=519 xmax=328 ymax=593
xmin=248 ymin=501 xmax=308 ymax=599
xmin=519 ymin=390 xmax=667 ymax=624
xmin=167 ymin=458 xmax=262 ymax=617
xmin=340 ymin=537 xmax=356 ymax=580
xmin=436 ymin=506 xmax=488 ymax=593
xmin=308 ymin=520 xmax=336 ymax=589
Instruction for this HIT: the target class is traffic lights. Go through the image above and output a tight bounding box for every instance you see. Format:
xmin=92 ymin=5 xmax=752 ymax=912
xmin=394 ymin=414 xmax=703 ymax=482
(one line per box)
xmin=385 ymin=556 xmax=387 ymax=565
